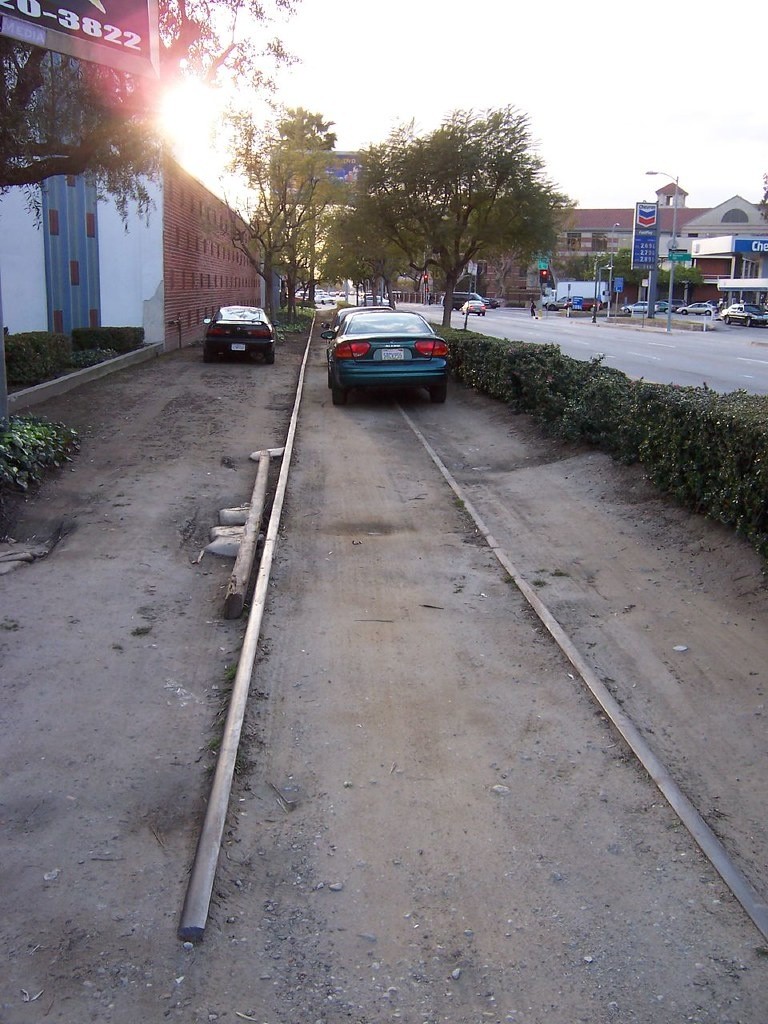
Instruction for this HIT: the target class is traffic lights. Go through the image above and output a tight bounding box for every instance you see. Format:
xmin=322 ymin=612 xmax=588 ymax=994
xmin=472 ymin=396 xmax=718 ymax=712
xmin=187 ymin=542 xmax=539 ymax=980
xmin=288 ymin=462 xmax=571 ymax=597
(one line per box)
xmin=423 ymin=274 xmax=428 ymax=282
xmin=539 ymin=268 xmax=548 ymax=283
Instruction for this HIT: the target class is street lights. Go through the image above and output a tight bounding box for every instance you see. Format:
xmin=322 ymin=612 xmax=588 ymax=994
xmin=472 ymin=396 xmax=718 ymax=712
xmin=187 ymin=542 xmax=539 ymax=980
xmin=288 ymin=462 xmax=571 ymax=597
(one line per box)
xmin=608 ymin=222 xmax=620 ymax=316
xmin=645 ymin=169 xmax=680 ymax=332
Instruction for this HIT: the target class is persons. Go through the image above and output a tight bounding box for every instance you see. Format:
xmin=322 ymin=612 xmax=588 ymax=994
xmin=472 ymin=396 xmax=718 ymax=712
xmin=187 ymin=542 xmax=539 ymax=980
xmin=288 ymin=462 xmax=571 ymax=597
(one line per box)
xmin=530 ymin=301 xmax=536 ymax=317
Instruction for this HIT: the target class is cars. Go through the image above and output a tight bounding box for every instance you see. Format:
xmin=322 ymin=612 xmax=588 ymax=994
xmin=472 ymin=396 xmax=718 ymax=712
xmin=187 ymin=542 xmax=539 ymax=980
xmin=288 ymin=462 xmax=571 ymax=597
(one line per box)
xmin=318 ymin=309 xmax=452 ymax=406
xmin=621 ymin=300 xmax=659 ymax=314
xmin=483 ymin=298 xmax=500 ymax=309
xmin=655 ymin=299 xmax=768 ymax=328
xmin=540 ymin=292 xmax=608 ymax=313
xmin=287 ymin=287 xmax=396 ymax=309
xmin=461 ymin=300 xmax=486 ymax=316
xmin=202 ymin=305 xmax=279 ymax=364
xmin=322 ymin=306 xmax=395 ymax=358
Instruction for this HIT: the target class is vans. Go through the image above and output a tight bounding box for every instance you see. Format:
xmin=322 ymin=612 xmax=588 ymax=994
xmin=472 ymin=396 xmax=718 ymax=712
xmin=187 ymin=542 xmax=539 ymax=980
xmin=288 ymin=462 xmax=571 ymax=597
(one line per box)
xmin=442 ymin=291 xmax=489 ymax=311
